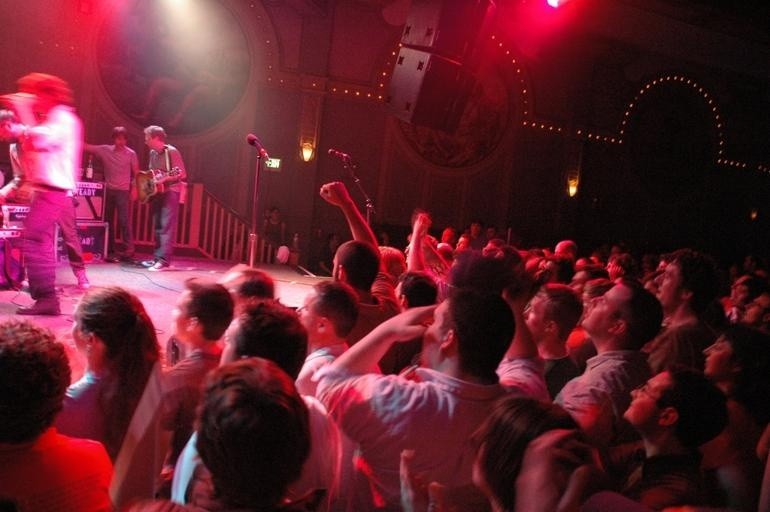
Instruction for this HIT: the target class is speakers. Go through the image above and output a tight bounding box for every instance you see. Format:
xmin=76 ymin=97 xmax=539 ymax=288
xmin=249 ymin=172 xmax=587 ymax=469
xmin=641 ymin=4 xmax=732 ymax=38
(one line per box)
xmin=71 ymin=183 xmax=105 ymax=220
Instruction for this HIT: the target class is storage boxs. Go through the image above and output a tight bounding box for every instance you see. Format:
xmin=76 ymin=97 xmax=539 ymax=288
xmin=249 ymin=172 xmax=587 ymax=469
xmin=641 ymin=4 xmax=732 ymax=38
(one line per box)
xmin=54 ymin=221 xmax=111 ymax=263
xmin=74 ymin=181 xmax=109 ymax=221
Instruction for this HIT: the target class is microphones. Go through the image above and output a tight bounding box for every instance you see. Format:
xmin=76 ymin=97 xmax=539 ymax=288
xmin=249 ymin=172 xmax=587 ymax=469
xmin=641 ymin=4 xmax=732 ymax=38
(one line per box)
xmin=246 ymin=132 xmax=269 ymax=161
xmin=326 ymin=149 xmax=348 ymax=159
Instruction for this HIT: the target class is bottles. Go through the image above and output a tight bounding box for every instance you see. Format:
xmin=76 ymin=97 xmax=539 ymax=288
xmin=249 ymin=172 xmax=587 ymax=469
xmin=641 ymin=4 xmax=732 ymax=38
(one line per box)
xmin=292 ymin=233 xmax=301 ymax=249
xmin=84 ymin=152 xmax=95 ymax=180
xmin=316 ymin=225 xmax=324 ymax=239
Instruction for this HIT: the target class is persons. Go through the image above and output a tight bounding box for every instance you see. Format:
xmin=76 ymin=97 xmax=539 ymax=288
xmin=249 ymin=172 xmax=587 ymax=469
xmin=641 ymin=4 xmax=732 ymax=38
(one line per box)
xmin=0 ymin=71 xmax=92 ymax=315
xmin=1 ymin=178 xmax=770 ymax=511
xmin=2 ymin=111 xmax=89 ymax=292
xmin=139 ymin=127 xmax=186 ymax=271
xmin=81 ymin=124 xmax=140 ymax=266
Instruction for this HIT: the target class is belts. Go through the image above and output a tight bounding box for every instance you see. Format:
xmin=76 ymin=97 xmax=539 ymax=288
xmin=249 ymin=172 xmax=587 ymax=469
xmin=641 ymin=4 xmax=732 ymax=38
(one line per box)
xmin=31 ymin=183 xmax=75 ymax=197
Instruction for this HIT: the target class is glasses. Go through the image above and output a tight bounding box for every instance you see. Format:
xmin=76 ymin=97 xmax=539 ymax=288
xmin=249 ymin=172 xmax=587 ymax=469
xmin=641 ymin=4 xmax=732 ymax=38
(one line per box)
xmin=633 ymin=381 xmax=661 ymax=402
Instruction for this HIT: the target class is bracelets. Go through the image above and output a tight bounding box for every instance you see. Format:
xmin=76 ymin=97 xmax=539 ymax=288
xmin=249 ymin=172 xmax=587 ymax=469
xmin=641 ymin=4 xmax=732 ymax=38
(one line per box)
xmin=20 ymin=124 xmax=31 ymax=143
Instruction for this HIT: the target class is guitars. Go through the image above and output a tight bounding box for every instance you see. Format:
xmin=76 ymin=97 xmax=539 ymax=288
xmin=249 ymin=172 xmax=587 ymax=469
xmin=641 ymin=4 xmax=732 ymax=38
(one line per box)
xmin=136 ymin=167 xmax=181 ymax=205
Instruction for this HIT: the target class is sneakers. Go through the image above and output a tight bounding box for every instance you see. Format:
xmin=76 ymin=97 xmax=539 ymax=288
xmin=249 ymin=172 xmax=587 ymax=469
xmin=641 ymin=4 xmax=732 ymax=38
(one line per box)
xmin=77 ymin=272 xmax=90 ymax=286
xmin=141 ymin=259 xmax=169 ymax=272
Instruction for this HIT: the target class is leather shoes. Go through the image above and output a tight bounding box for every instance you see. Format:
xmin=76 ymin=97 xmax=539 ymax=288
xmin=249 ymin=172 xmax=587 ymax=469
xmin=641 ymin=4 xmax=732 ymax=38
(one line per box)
xmin=16 ymin=298 xmax=61 ymax=315
